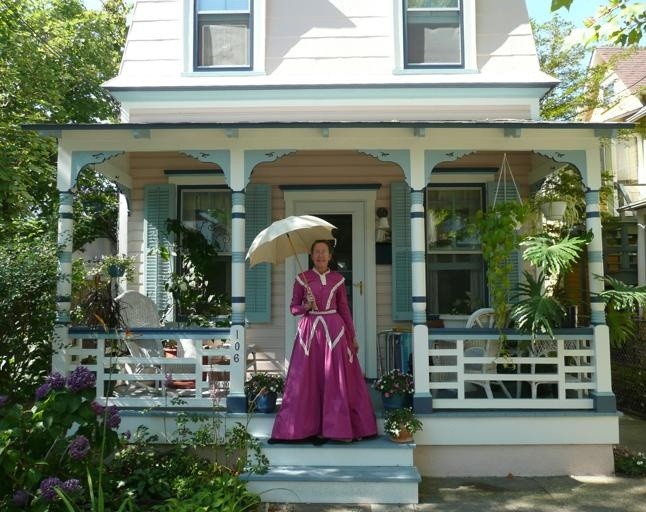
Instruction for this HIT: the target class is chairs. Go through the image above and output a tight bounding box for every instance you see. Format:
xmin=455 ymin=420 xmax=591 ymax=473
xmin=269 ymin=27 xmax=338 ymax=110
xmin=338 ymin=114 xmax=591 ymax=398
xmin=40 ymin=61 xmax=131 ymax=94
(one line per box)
xmin=111 ymin=290 xmax=196 ymax=397
xmin=430 ymin=308 xmax=513 ymax=398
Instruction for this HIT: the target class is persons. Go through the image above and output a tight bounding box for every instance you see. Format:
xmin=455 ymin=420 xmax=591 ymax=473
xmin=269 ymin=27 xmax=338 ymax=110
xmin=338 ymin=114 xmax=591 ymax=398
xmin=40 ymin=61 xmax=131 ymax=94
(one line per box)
xmin=268 ymin=240 xmax=379 ymax=446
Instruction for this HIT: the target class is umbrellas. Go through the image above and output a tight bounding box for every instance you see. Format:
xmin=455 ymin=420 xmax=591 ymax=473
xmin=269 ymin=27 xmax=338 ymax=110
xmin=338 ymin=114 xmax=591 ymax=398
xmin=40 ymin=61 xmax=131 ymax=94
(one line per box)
xmin=244 ymin=214 xmax=339 ymax=312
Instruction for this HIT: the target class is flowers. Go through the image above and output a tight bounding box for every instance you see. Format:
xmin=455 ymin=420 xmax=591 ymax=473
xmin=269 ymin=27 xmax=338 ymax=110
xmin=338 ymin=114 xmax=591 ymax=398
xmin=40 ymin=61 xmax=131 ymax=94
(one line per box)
xmin=91 ymin=253 xmax=135 ymax=282
xmin=244 ymin=372 xmax=285 ymax=407
xmin=372 ymin=368 xmax=415 ymax=399
xmin=384 ymin=406 xmax=424 ymax=438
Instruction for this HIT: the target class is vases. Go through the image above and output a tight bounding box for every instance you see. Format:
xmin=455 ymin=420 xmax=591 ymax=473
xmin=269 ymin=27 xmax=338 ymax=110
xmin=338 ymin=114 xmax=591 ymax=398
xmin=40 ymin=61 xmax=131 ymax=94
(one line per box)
xmin=254 ymin=393 xmax=276 ymax=412
xmin=381 ymin=391 xmax=408 ymax=411
xmin=108 ymin=266 xmax=125 ymax=277
xmin=388 ymin=422 xmax=414 ymax=442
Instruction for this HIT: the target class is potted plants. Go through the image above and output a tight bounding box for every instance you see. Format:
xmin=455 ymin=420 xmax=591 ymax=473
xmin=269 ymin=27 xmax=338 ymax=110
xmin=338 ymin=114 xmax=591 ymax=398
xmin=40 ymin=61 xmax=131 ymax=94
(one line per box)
xmin=147 ymin=211 xmax=232 ymax=389
xmin=467 ymin=198 xmax=549 ymax=329
xmin=533 ymin=164 xmax=587 ymax=231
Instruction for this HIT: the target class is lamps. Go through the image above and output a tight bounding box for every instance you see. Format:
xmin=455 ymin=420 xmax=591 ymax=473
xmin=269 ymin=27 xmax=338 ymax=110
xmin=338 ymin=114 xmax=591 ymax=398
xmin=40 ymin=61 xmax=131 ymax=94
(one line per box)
xmin=376 ymin=208 xmax=390 ymax=242
